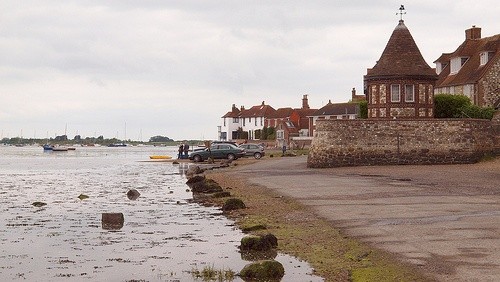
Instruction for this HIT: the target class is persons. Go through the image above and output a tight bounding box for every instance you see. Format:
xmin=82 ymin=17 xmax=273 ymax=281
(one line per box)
xmin=178 ymin=143 xmax=184 ymax=160
xmin=184 ymin=142 xmax=189 ymax=159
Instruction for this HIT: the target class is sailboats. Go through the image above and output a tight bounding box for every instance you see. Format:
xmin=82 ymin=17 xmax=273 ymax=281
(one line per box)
xmin=41 ymin=125 xmax=76 ymax=151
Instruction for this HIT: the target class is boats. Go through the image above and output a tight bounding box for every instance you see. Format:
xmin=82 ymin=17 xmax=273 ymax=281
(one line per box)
xmin=149 ymin=155 xmax=172 ymax=159
xmin=107 ymin=142 xmax=128 ymax=147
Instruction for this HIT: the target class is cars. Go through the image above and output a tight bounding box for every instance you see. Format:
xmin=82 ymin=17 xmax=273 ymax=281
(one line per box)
xmin=188 ymin=143 xmax=246 ymax=163
xmin=236 ymin=142 xmax=265 ymax=160
